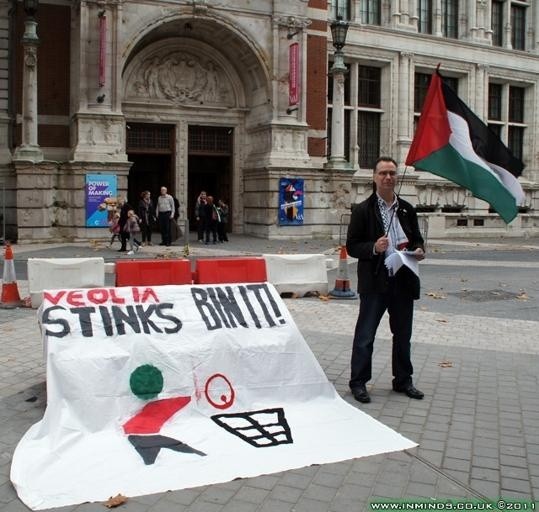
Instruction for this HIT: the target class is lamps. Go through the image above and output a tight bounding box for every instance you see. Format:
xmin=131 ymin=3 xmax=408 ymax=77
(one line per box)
xmin=328 ymin=2 xmax=349 ymax=73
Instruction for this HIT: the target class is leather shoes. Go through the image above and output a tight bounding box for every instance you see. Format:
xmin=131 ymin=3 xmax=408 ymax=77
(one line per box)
xmin=350 ymin=382 xmax=372 ymax=404
xmin=390 ymin=379 xmax=426 ymax=399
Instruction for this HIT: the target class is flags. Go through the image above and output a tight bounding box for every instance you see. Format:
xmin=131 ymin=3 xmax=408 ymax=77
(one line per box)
xmin=405 ymin=70 xmax=527 ymax=225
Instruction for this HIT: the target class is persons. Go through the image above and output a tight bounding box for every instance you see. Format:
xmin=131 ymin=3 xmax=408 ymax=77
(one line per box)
xmin=347 ymin=157 xmax=425 ymax=403
xmin=106 ymin=186 xmax=180 ymax=255
xmin=195 ymin=191 xmax=229 ymax=243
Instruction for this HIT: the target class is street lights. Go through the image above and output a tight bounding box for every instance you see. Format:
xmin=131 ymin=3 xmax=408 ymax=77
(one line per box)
xmin=327 ymin=14 xmax=350 ymax=161
xmin=17 ymin=0 xmax=43 ymax=148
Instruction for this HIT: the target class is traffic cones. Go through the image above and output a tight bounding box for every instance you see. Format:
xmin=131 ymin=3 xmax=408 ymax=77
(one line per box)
xmin=329 ymin=245 xmax=355 ymax=297
xmin=0 ymin=243 xmax=22 ymax=309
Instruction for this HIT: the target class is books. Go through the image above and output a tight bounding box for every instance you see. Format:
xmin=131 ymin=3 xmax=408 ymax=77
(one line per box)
xmin=384 ymin=250 xmax=422 ymax=277
